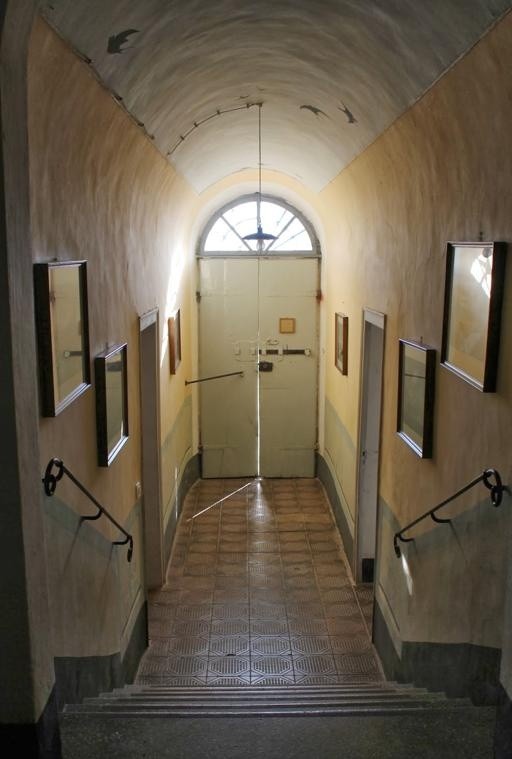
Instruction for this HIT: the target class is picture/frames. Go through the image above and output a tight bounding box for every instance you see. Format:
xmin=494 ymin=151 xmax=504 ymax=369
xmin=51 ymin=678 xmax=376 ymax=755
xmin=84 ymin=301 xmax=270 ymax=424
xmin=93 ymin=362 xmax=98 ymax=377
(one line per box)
xmin=95 ymin=342 xmax=132 ymax=467
xmin=334 ymin=313 xmax=349 ymax=375
xmin=168 ymin=307 xmax=181 ymax=376
xmin=439 ymin=241 xmax=504 ymax=394
xmin=397 ymin=339 xmax=437 ymax=458
xmin=32 ymin=259 xmax=92 ymax=418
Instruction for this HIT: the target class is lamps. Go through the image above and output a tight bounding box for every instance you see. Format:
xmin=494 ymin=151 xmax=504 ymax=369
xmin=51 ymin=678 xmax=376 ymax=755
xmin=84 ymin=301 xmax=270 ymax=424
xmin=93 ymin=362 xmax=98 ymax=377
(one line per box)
xmin=239 ymin=103 xmax=279 ymax=255
